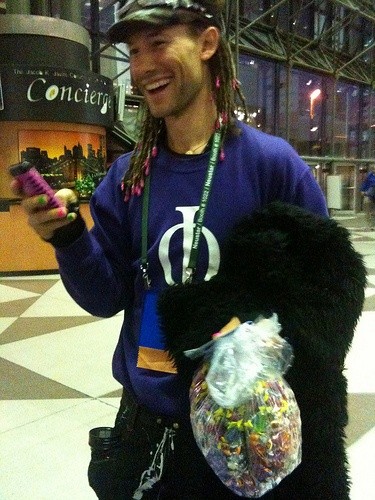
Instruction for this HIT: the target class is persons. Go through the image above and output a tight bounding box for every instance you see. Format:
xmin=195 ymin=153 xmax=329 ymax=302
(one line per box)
xmin=360 ymin=163 xmax=375 ymax=231
xmin=9 ymin=0 xmax=331 ymax=500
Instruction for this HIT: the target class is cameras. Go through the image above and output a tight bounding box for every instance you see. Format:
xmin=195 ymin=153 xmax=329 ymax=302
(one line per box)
xmin=86 ymin=426 xmax=137 ymax=499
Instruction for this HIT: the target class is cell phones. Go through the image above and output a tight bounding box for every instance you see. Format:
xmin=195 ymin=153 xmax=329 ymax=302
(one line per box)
xmin=7 ymin=161 xmax=61 ymax=212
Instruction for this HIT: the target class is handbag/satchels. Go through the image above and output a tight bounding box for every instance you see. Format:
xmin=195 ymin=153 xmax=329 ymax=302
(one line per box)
xmin=187 ymin=318 xmax=303 ymax=499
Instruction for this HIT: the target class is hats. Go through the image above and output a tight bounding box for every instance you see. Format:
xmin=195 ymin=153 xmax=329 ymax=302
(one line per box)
xmin=101 ymin=0 xmax=226 ymax=44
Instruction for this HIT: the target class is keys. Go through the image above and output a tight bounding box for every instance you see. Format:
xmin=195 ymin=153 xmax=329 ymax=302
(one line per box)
xmin=156 ymin=427 xmax=176 ymax=461
